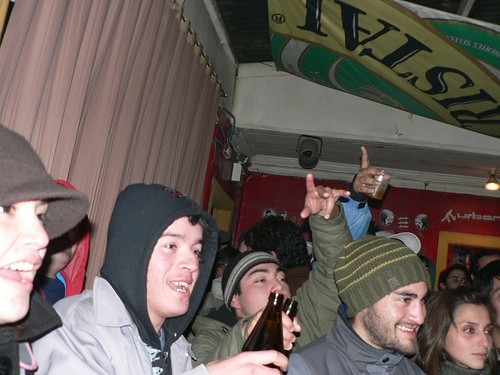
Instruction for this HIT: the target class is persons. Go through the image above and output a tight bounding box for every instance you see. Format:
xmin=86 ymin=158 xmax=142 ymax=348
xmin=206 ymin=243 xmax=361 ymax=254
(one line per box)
xmin=186 ymin=172 xmax=354 ymax=368
xmin=416 ymin=286 xmax=498 ymax=375
xmin=199 ymin=146 xmax=500 ymax=360
xmin=287 ymin=236 xmax=427 ymax=375
xmin=0 ymin=123 xmax=89 ymax=375
xmin=32 ymin=183 xmax=289 ymax=375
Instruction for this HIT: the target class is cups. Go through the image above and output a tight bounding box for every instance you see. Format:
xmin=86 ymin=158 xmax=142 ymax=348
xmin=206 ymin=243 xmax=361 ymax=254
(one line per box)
xmin=365 ymin=169 xmax=393 ymax=200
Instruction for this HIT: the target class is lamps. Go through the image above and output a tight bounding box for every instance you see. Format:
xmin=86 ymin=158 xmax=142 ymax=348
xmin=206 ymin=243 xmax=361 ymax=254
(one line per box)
xmin=296 ymin=134 xmax=324 ymax=169
xmin=484 ymin=168 xmax=499 ymax=191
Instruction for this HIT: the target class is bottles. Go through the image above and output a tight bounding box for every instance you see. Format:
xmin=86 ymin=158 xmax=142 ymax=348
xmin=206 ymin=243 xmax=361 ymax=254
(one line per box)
xmin=280 ymin=300 xmax=298 ymax=375
xmin=242 ymin=293 xmax=285 ymax=369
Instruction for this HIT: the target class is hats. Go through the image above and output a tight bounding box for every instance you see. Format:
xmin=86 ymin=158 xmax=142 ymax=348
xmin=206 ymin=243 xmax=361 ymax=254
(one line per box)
xmin=222 ymin=249 xmax=285 ymax=313
xmin=0 ymin=122 xmax=90 ymax=240
xmin=334 ymin=236 xmax=431 ymax=317
xmin=375 ymin=230 xmax=421 ymax=255
